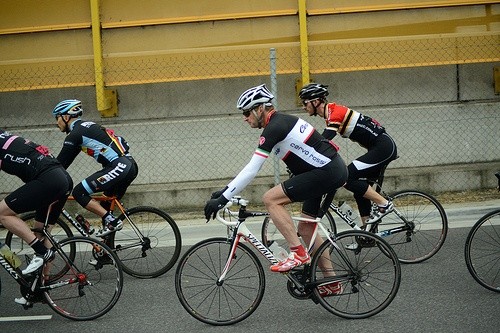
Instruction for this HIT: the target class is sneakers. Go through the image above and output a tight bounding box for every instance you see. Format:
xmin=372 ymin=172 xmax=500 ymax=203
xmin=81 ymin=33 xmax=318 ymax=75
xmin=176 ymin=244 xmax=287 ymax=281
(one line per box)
xmin=14 ymin=291 xmax=51 ymax=305
xmin=21 ymin=249 xmax=55 ymax=274
xmin=344 ymin=236 xmax=376 ymax=250
xmin=270 ymin=247 xmax=311 ymax=272
xmin=365 ymin=199 xmax=393 ymax=224
xmin=317 ymin=281 xmax=343 ymax=297
xmin=88 ymin=253 xmax=114 ymax=266
xmin=95 ymin=211 xmax=123 ymax=237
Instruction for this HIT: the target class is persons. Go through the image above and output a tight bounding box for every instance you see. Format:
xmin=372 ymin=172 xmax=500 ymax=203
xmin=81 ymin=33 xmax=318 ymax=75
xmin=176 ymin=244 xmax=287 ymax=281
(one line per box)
xmin=202 ymin=82 xmax=349 ymax=297
xmin=297 ymin=83 xmax=397 ymax=252
xmin=41 ymin=99 xmax=138 ymax=268
xmin=0 ymin=128 xmax=74 ymax=305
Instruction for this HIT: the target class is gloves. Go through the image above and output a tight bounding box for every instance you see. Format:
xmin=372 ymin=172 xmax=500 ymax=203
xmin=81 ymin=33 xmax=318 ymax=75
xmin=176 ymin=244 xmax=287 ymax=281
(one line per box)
xmin=210 ymin=186 xmax=229 ymax=199
xmin=203 ymin=194 xmax=229 ymax=223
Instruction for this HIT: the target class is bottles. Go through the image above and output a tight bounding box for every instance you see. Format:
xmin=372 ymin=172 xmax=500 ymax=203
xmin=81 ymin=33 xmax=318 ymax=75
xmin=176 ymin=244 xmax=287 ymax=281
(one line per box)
xmin=338 ymin=200 xmax=360 ymax=221
xmin=267 ymin=240 xmax=288 ymax=263
xmin=73 ymin=210 xmax=94 ymax=235
xmin=0 ymin=242 xmax=22 ymax=268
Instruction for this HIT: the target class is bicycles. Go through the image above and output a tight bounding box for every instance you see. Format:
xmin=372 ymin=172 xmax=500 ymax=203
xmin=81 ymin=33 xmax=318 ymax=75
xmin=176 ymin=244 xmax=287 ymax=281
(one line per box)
xmin=174 ymin=193 xmax=401 ymax=327
xmin=5 ymin=183 xmax=182 ymax=279
xmin=465 ymin=170 xmax=500 ymax=293
xmin=261 ymin=152 xmax=448 ymax=264
xmin=0 ymin=190 xmax=124 ymax=321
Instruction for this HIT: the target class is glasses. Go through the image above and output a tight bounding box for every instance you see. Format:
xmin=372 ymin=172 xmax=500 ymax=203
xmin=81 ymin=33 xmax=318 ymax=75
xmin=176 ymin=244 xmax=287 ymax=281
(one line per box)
xmin=243 ymin=103 xmax=261 ymax=118
xmin=302 ymin=98 xmax=320 ymax=107
xmin=55 ymin=115 xmax=71 ymax=121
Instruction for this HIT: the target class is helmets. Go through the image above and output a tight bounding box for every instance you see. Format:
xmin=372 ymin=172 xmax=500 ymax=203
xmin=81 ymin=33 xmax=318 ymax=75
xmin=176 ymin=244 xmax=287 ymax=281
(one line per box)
xmin=52 ymin=99 xmax=83 ymax=117
xmin=298 ymin=82 xmax=329 ymax=99
xmin=236 ymin=83 xmax=274 ymax=112
